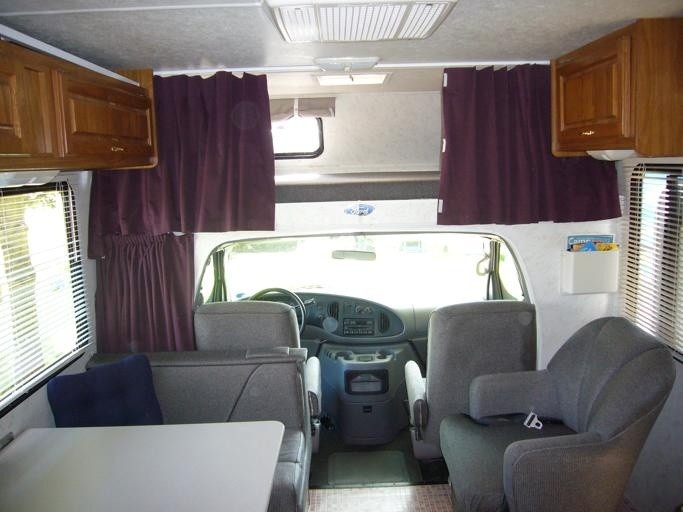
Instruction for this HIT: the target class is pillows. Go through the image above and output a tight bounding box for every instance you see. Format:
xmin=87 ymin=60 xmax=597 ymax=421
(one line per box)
xmin=48 ymin=354 xmax=163 ymax=428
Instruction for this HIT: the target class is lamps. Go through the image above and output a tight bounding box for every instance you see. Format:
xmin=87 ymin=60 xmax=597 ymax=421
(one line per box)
xmin=0 ymin=171 xmax=58 ymax=188
xmin=584 ymin=150 xmax=634 ymax=161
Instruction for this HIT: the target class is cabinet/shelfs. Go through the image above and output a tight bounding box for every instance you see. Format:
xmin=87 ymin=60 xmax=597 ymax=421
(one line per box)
xmin=0 ymin=40 xmax=157 ymax=172
xmin=548 ymin=17 xmax=681 ymax=162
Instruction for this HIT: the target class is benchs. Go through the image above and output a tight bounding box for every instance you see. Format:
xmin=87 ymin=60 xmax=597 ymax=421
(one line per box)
xmin=91 ymin=352 xmax=311 ymax=511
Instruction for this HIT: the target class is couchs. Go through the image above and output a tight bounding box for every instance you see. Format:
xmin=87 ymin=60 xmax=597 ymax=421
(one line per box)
xmin=439 ymin=316 xmax=677 ymax=511
xmin=192 ymin=299 xmax=323 ymax=450
xmin=406 ymin=299 xmax=536 ymax=471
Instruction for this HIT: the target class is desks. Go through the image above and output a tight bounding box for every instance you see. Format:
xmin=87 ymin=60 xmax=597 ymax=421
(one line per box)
xmin=0 ymin=415 xmax=285 ymax=511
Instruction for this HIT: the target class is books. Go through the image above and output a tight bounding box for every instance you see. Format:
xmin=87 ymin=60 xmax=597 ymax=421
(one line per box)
xmin=567 ymin=235 xmax=620 ymax=252
